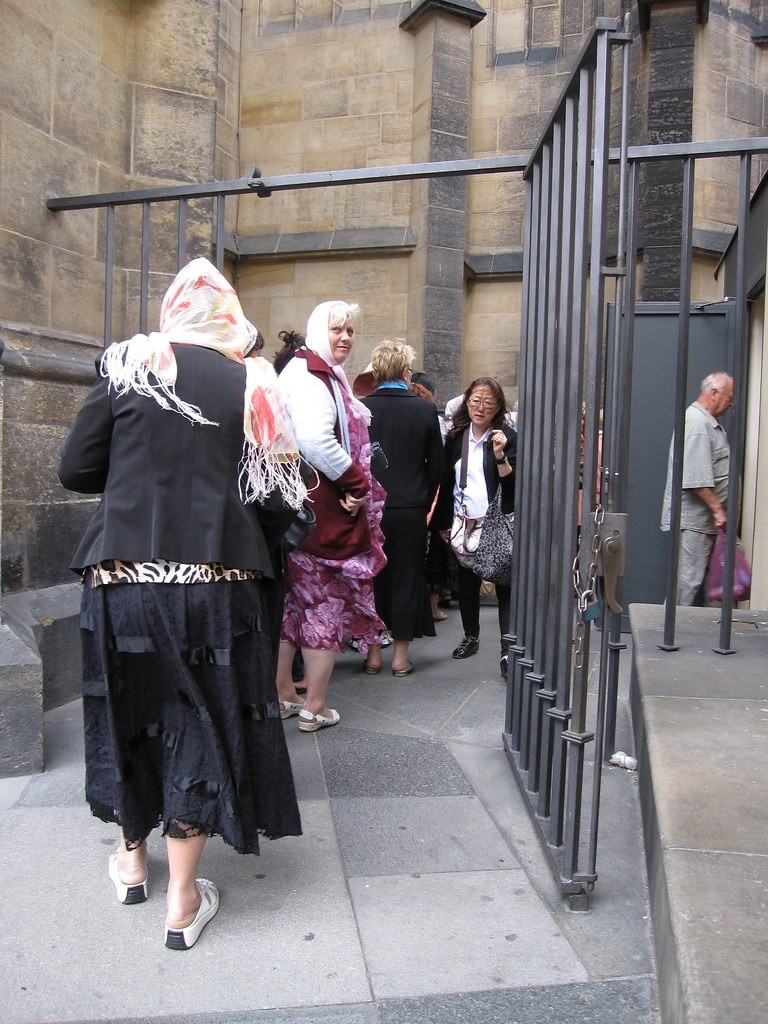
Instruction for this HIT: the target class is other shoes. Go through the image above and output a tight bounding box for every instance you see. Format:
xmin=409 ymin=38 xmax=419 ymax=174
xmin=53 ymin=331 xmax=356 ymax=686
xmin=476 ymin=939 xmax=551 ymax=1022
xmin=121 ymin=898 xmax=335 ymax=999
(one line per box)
xmin=436 ymin=592 xmax=453 ymax=609
xmin=432 ymin=611 xmax=448 ymax=620
xmin=294 ymin=686 xmax=307 ymax=695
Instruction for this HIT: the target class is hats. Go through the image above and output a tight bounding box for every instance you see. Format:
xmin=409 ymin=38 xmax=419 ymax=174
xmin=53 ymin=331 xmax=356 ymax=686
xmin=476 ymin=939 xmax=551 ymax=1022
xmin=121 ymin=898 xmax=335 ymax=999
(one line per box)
xmin=362 ymin=362 xmax=374 ymax=373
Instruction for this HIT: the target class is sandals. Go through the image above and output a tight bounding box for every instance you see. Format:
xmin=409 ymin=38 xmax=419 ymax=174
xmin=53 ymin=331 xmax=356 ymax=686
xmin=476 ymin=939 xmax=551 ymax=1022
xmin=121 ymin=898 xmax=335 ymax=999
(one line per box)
xmin=279 ymin=700 xmax=306 ymax=720
xmin=298 ymin=708 xmax=340 ymax=732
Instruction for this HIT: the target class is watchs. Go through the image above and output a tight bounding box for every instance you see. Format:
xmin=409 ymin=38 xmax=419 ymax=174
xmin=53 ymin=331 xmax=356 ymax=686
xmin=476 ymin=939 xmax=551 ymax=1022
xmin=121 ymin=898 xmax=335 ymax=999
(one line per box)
xmin=495 ymin=454 xmax=508 ymax=464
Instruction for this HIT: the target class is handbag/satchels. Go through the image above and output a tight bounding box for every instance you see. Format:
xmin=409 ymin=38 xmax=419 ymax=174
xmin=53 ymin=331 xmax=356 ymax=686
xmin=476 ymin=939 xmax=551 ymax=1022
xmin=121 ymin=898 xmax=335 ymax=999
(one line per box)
xmin=473 ymin=483 xmax=515 ymax=586
xmin=706 ymin=529 xmax=751 ymax=601
xmin=252 ymin=450 xmax=318 ymax=552
xmin=450 ymin=504 xmax=486 ymax=568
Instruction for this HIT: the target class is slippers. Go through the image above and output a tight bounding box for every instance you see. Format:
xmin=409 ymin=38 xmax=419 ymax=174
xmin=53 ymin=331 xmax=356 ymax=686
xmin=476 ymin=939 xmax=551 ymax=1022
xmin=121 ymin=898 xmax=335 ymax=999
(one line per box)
xmin=165 ymin=878 xmax=219 ymax=950
xmin=108 ymin=847 xmax=148 ymax=904
xmin=363 ymin=659 xmax=378 ymax=675
xmin=394 ymin=661 xmax=415 ymax=677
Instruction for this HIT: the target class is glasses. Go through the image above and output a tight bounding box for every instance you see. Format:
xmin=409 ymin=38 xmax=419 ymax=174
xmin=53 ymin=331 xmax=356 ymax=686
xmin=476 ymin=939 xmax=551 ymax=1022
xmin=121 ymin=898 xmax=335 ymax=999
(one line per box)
xmin=408 ymin=368 xmax=414 ymax=374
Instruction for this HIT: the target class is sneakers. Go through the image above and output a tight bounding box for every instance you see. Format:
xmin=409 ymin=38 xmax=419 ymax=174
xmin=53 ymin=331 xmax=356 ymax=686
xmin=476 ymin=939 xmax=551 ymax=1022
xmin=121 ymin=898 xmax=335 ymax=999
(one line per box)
xmin=453 ymin=636 xmax=479 ymax=658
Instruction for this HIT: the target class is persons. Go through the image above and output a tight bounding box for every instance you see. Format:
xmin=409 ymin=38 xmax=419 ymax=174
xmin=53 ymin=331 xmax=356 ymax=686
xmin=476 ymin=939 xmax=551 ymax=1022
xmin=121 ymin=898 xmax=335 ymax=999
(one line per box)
xmin=276 ymin=300 xmax=388 ymax=732
xmin=243 ymin=330 xmax=608 ymax=693
xmin=660 ymin=371 xmax=741 ymax=607
xmin=57 ymin=257 xmax=314 ymax=950
